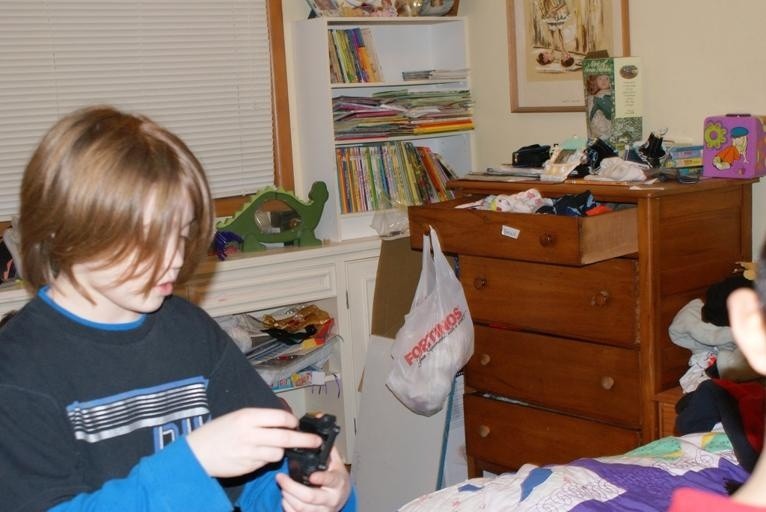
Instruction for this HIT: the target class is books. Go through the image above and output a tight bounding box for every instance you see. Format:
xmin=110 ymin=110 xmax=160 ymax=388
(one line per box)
xmin=329 ymin=26 xmax=472 ymax=82
xmin=330 ymin=85 xmax=476 ymax=141
xmin=335 ymin=140 xmax=460 ymax=215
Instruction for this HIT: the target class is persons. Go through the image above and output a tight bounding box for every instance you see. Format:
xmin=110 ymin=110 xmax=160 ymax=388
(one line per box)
xmin=586 ymin=73 xmax=613 ymax=128
xmin=0 ymin=106 xmax=357 ymax=512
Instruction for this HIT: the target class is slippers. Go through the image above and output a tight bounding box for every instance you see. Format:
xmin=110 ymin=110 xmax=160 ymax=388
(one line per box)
xmin=560 ymin=53 xmax=574 ymax=68
xmin=535 ymin=51 xmax=556 ymax=65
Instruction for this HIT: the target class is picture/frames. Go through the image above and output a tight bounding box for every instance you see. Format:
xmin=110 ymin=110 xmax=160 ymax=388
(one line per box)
xmin=506 ymin=0 xmax=629 ymax=114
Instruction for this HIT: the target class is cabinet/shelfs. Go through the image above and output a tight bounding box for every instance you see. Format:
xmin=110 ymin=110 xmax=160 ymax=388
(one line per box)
xmin=295 ymin=17 xmax=475 ymax=239
xmin=1 ymin=235 xmax=408 ymax=466
xmin=408 ymin=176 xmax=761 ymax=478
